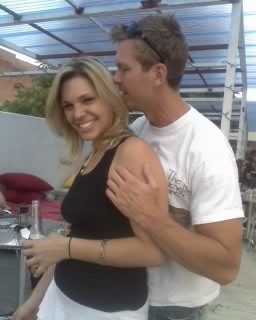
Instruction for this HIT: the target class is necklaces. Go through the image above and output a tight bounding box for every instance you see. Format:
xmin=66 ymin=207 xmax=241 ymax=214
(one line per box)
xmin=79 ymin=138 xmax=117 ymax=173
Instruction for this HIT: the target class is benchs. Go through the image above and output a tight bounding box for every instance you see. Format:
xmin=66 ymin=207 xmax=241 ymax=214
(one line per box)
xmin=6 ymin=200 xmax=64 ymax=222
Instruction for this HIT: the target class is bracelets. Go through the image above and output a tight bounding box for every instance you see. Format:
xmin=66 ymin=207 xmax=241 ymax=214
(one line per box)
xmin=67 ymin=236 xmax=73 ymax=262
xmin=99 ymin=238 xmax=107 ymax=266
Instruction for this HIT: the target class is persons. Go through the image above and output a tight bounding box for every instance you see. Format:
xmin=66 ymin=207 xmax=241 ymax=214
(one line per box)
xmin=103 ymin=12 xmax=247 ymax=320
xmin=7 ymin=56 xmax=168 ymax=320
xmin=0 ymin=191 xmax=11 ymax=218
xmin=236 ymin=149 xmax=256 ymax=229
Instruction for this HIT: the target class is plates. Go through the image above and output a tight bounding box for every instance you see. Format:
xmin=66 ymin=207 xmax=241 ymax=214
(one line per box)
xmin=0 ymin=222 xmax=12 ymax=228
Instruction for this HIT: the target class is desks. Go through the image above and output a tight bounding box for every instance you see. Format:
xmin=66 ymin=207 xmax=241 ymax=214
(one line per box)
xmin=0 ymin=217 xmax=66 ymax=320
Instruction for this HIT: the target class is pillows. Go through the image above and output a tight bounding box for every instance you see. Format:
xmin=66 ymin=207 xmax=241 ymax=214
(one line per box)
xmin=0 ymin=173 xmax=54 ymax=203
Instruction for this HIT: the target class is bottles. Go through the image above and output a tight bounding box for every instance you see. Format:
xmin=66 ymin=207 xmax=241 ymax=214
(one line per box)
xmin=28 ymin=200 xmax=48 ymax=273
xmin=19 ymin=207 xmax=28 ymax=227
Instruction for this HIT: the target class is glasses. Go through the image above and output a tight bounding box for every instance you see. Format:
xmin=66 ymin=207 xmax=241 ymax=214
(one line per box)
xmin=121 ymin=20 xmax=165 ymax=63
